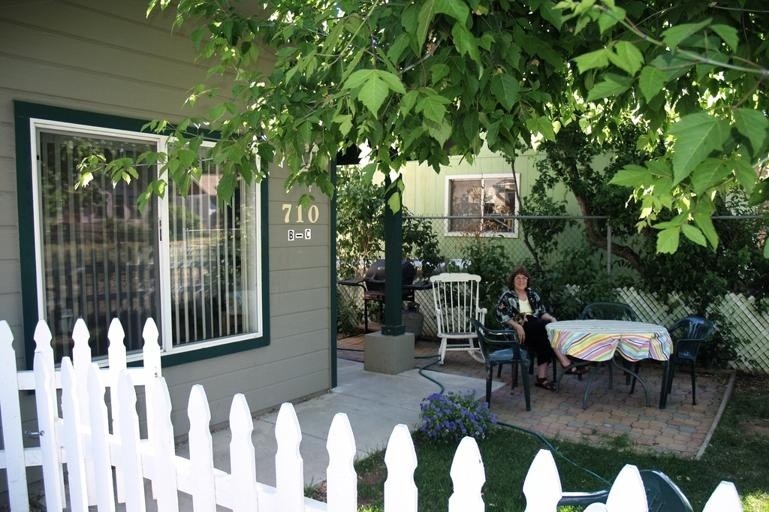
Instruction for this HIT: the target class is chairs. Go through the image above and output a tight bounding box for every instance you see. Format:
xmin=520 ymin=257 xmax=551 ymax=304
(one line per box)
xmin=558 ymin=469 xmax=695 ymax=512
xmin=429 ymin=272 xmax=715 ymax=411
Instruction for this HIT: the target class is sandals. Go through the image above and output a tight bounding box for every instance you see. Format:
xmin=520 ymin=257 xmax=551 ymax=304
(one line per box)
xmin=564 ymin=362 xmax=589 ymax=375
xmin=534 ymin=375 xmax=556 ymax=390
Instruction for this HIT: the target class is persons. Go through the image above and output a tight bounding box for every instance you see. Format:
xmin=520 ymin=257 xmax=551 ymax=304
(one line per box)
xmin=494 ymin=267 xmax=592 ymax=392
